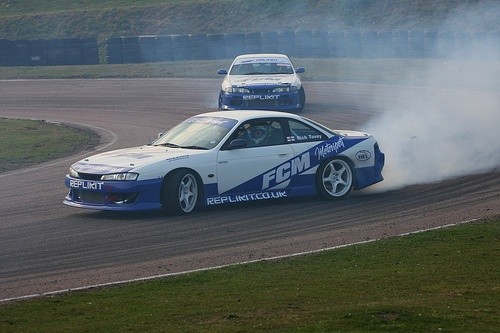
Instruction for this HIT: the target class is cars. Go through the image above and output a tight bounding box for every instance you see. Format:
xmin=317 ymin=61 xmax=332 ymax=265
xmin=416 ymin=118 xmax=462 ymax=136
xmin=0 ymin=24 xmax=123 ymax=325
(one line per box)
xmin=217 ymin=53 xmax=306 ymax=115
xmin=62 ymin=110 xmax=385 ymax=215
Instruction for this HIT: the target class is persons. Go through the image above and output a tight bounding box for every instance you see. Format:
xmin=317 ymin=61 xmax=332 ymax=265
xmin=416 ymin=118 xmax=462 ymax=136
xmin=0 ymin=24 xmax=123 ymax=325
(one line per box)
xmin=249 ymin=121 xmax=272 ymax=145
